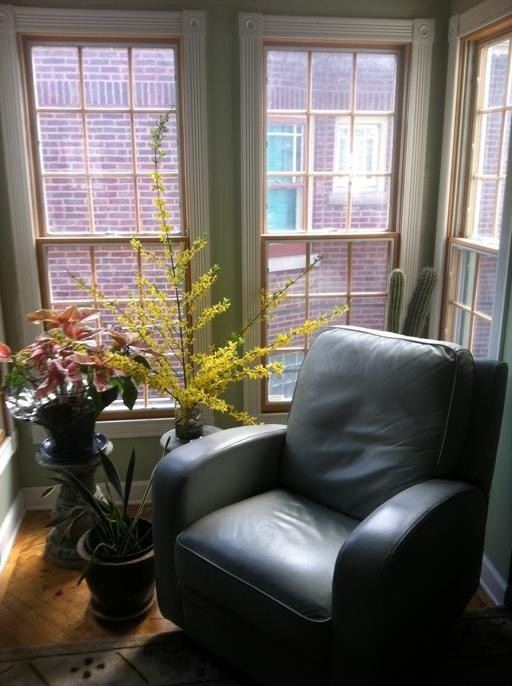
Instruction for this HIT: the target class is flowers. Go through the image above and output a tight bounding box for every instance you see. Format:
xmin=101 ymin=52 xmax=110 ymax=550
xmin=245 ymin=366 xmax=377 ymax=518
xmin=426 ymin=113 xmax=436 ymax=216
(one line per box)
xmin=0 ymin=307 xmax=154 ymax=419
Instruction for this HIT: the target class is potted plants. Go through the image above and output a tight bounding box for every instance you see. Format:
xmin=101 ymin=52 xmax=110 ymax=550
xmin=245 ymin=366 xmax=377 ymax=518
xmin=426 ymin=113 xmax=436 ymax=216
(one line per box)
xmin=39 ymin=437 xmax=162 ymax=618
xmin=30 ymin=103 xmax=349 ymax=425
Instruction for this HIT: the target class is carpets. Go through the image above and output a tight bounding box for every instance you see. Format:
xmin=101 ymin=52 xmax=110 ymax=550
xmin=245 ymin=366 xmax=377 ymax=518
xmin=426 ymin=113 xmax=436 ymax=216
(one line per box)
xmin=0 ymin=606 xmax=512 ymax=685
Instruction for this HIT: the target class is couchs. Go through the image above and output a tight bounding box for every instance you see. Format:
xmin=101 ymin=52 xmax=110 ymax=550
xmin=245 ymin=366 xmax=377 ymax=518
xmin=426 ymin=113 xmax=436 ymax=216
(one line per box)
xmin=153 ymin=323 xmax=509 ymax=685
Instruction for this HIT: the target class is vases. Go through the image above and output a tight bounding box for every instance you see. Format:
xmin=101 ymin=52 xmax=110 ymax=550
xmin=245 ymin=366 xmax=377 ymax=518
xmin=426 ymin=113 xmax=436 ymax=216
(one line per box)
xmin=174 ymin=398 xmax=202 ymax=441
xmin=33 ymin=399 xmax=104 ymax=460
